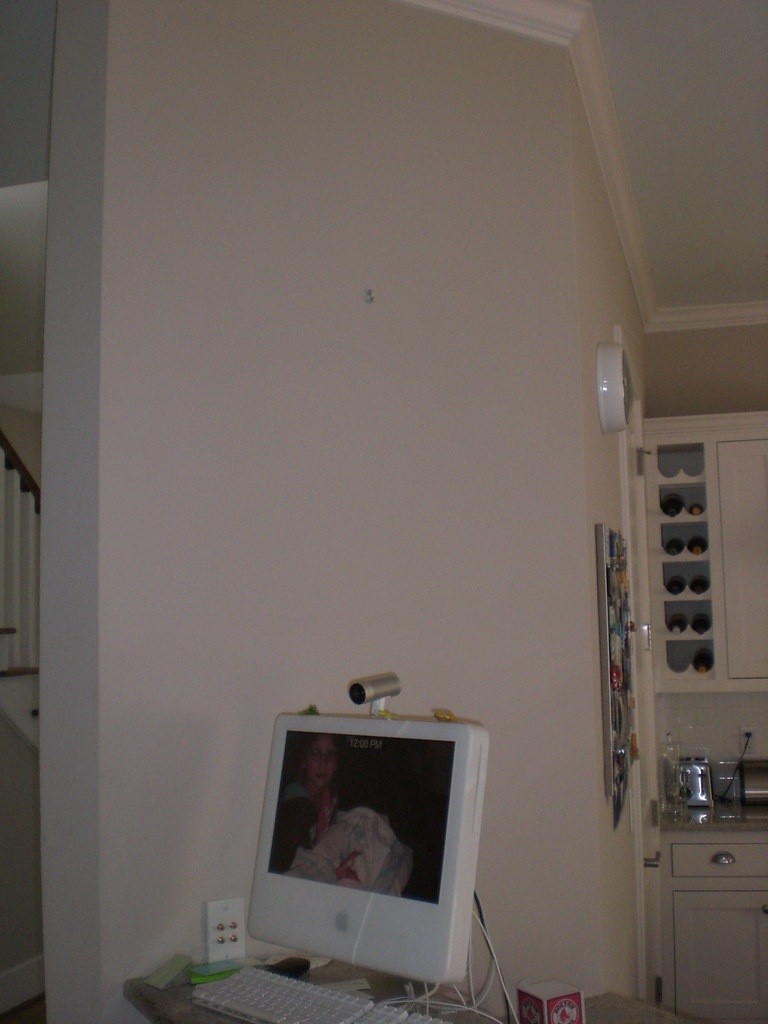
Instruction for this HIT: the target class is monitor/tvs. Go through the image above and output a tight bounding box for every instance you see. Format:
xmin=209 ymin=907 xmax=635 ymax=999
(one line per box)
xmin=247 ymin=711 xmax=488 ymax=983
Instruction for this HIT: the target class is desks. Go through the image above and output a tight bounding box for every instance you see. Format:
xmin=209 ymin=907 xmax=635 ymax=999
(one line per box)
xmin=122 ymin=958 xmax=502 ymax=1023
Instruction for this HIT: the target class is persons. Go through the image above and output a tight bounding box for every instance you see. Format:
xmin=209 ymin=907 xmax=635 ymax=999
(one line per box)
xmin=268 ymin=732 xmax=415 ymax=899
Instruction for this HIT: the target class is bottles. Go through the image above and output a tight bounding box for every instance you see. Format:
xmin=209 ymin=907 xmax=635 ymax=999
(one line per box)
xmin=667 ymin=575 xmax=685 ymax=595
xmin=687 ymin=536 xmax=707 ymax=555
xmin=689 ymin=503 xmax=704 ymax=516
xmin=661 ymin=492 xmax=684 ymax=517
xmin=667 ymin=613 xmax=688 ymax=633
xmin=693 ymin=648 xmax=714 ymax=673
xmin=680 ymin=753 xmax=710 ymax=801
xmin=665 ymin=538 xmax=685 ymax=555
xmin=691 ymin=614 xmax=711 ymax=634
xmin=689 ymin=575 xmax=710 ymax=594
xmin=656 ymin=731 xmax=687 ymax=816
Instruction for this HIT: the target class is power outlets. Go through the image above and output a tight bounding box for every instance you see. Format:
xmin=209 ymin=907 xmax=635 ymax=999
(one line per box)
xmin=739 ymin=727 xmax=757 ymax=755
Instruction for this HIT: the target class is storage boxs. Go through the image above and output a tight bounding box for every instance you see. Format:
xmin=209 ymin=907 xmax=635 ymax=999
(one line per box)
xmin=516 ymin=978 xmax=585 ymax=1024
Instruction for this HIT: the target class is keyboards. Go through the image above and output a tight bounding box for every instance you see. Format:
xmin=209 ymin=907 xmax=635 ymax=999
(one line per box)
xmin=190 ymin=965 xmax=447 ymax=1024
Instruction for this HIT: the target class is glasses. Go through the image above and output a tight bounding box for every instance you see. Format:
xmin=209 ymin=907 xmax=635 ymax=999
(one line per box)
xmin=304 ymin=748 xmax=338 ymax=762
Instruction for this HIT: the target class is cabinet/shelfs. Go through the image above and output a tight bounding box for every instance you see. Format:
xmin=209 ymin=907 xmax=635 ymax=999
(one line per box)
xmin=659 ymin=830 xmax=767 ymax=1023
xmin=716 ymin=440 xmax=767 ymax=679
xmin=653 ymin=440 xmax=717 ymax=680
